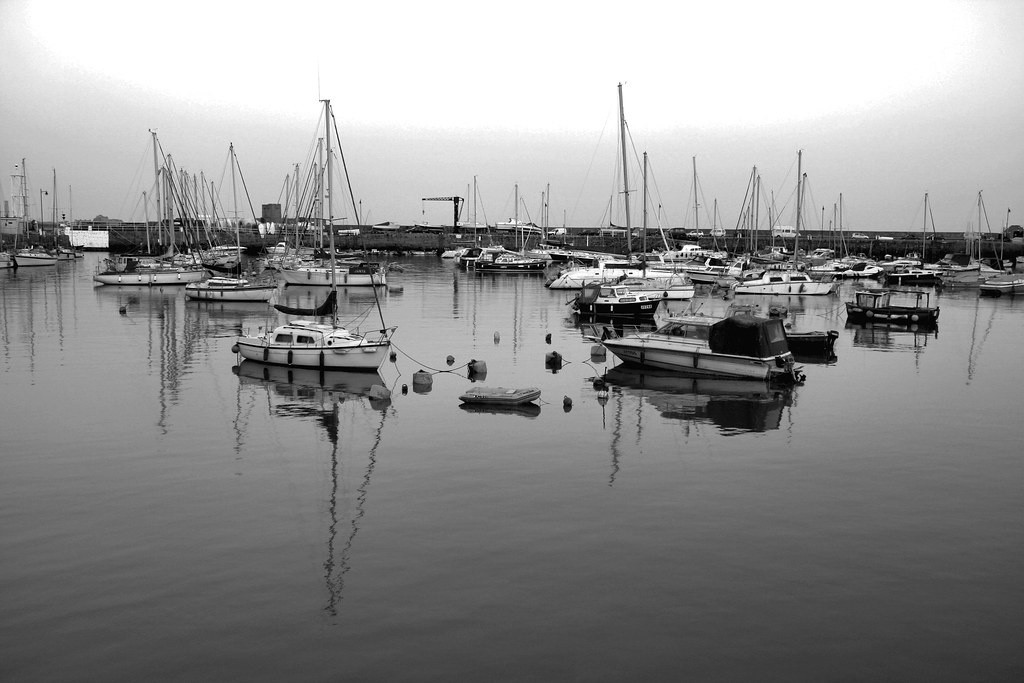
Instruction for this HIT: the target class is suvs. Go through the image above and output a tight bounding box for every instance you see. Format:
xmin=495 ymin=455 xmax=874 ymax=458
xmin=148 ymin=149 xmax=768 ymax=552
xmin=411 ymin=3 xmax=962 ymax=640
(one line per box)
xmin=548 ymin=228 xmax=567 ymax=236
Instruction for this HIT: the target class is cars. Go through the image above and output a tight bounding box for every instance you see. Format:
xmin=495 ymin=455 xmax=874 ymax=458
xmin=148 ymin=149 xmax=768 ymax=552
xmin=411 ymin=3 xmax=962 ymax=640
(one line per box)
xmin=685 ymin=229 xmax=705 ymax=239
xmin=576 ymin=229 xmax=596 ymax=236
xmin=709 ymin=229 xmax=727 ymax=238
xmin=852 ymin=231 xmax=869 ymax=239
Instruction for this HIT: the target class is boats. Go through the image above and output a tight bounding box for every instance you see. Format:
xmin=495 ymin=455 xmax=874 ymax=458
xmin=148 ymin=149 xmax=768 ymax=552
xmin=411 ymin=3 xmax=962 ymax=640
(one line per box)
xmin=459 ymin=385 xmax=542 ymax=406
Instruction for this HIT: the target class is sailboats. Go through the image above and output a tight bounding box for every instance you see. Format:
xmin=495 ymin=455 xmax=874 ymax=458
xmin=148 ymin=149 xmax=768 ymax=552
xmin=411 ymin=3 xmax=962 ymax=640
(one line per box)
xmin=438 ymin=81 xmax=1024 ymax=386
xmin=0 ymin=127 xmax=407 ymax=303
xmin=235 ymin=98 xmax=400 ymax=370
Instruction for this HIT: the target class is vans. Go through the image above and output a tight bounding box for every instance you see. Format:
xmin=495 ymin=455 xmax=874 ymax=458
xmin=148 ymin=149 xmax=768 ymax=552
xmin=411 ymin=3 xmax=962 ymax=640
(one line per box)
xmin=772 ymin=226 xmax=801 ymax=238
